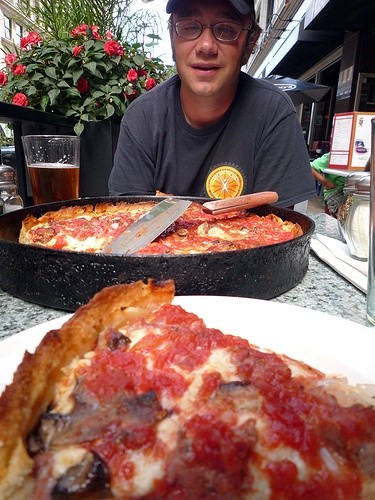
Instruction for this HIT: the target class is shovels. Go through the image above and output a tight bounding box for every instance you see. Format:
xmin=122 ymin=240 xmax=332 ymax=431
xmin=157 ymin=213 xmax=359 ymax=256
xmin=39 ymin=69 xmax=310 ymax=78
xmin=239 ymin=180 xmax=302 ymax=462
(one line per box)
xmin=101 ymin=191 xmax=279 ymax=254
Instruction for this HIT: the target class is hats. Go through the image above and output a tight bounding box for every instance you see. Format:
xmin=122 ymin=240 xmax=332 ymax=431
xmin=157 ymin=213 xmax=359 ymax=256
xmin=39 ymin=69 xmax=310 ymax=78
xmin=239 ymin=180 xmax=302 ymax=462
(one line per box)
xmin=166 ymin=1 xmax=256 ymax=22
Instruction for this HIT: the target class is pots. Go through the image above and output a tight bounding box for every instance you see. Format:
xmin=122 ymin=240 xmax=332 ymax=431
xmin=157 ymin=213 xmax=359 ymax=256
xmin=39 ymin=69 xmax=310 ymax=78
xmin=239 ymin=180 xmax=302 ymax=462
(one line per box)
xmin=0 ymin=194 xmax=316 ymax=313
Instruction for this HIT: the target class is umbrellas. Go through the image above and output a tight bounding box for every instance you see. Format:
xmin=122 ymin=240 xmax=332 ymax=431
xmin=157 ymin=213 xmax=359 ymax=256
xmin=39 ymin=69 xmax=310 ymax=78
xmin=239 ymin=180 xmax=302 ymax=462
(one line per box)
xmin=260 ymin=74 xmax=333 ymax=106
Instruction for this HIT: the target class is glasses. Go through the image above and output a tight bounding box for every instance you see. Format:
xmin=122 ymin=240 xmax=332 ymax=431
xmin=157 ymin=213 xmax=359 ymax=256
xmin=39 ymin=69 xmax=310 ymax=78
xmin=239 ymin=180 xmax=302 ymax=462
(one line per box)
xmin=170 ymin=18 xmax=252 ymax=43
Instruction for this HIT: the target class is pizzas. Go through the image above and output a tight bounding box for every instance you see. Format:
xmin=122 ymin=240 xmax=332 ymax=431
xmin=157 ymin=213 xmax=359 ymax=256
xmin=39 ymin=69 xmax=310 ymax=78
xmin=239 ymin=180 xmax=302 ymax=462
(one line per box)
xmin=18 ymin=201 xmax=303 ymax=257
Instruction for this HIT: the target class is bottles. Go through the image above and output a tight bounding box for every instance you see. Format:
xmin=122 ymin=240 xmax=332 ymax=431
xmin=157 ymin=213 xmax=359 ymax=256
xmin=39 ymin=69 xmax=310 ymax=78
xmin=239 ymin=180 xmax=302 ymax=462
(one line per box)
xmin=0 ymin=164 xmax=24 ymax=216
xmin=344 ymin=175 xmax=371 ymax=262
xmin=337 ymin=172 xmax=370 ymax=244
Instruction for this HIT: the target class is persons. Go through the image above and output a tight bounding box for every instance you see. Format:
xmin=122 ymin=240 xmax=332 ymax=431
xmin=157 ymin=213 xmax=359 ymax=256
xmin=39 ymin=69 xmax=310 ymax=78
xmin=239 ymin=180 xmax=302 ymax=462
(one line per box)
xmin=310 ymin=152 xmax=347 ymax=218
xmin=107 ymin=0 xmax=318 ymax=209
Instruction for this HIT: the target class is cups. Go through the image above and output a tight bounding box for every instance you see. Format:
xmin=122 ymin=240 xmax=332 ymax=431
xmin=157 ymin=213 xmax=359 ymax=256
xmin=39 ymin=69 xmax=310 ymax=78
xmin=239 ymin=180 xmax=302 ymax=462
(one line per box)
xmin=19 ymin=133 xmax=82 ymax=206
xmin=366 ymin=117 xmax=375 ymax=325
